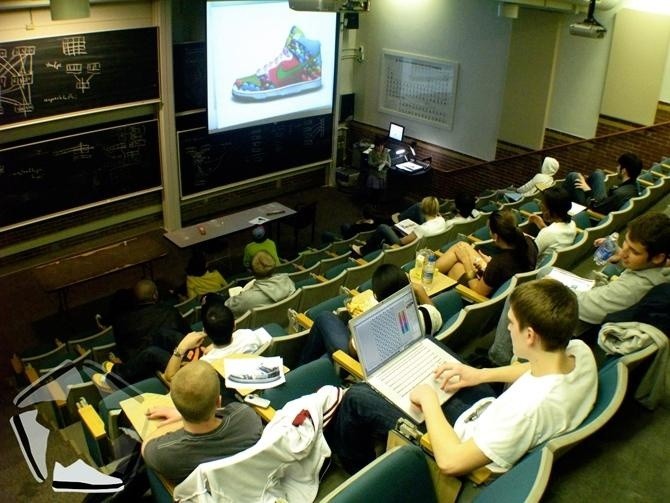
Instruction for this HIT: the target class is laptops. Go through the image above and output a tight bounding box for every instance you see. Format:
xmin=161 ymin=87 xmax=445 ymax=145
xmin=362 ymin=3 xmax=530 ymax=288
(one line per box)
xmin=349 ymin=283 xmax=463 ymax=425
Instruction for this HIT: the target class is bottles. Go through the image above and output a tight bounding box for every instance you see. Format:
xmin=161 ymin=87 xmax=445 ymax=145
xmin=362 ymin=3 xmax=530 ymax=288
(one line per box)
xmin=593 ymin=232 xmax=619 ymax=266
xmin=421 ymin=253 xmax=436 ymax=284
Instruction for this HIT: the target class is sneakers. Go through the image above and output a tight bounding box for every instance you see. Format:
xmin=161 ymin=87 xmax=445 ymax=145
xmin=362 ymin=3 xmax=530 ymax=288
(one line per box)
xmin=232 ymin=26 xmax=322 ymax=99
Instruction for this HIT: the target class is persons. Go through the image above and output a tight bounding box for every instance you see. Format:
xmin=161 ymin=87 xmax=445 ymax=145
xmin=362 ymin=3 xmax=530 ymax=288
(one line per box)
xmin=367 ymin=139 xmax=392 ymax=211
xmin=295 ymin=263 xmax=443 ymax=386
xmin=445 ymin=198 xmax=475 ymax=225
xmin=321 ymin=204 xmax=378 ymax=242
xmin=324 ymin=279 xmax=598 ymax=477
xmin=186 ymin=256 xmax=228 ymax=299
xmin=200 ymin=249 xmax=296 ymax=320
xmin=141 ymin=360 xmax=263 ymax=484
xmin=468 ymin=212 xmax=670 ymax=366
xmin=527 ymin=187 xmax=577 ymax=258
xmin=563 ymin=154 xmax=643 ymax=215
xmin=517 ymin=157 xmax=561 ymax=193
xmin=435 ymin=210 xmax=537 ymax=309
xmin=165 ymin=305 xmax=262 ymax=381
xmin=348 ymin=196 xmax=446 ymax=257
xmin=243 ymin=225 xmax=280 ymax=274
xmin=108 ymin=279 xmax=192 ymax=384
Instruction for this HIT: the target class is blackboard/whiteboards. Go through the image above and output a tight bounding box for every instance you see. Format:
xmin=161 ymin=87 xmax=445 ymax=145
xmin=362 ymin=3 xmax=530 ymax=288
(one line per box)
xmin=0 ymin=25 xmax=161 ymax=128
xmin=177 ymin=114 xmax=334 ymax=201
xmin=171 ymin=41 xmax=208 ymax=116
xmin=0 ymin=118 xmax=164 ymax=230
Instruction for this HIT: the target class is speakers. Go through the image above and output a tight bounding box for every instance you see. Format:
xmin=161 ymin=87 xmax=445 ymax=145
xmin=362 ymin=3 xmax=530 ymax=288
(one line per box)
xmin=343 ymin=12 xmax=360 ymax=29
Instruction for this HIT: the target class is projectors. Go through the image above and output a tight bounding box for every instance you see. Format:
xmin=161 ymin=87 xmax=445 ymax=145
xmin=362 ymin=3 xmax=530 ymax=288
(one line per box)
xmin=287 ymin=1 xmax=370 ymax=13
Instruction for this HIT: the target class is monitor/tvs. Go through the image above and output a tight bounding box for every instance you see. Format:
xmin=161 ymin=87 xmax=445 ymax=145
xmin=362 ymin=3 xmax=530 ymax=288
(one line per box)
xmin=388 ymin=122 xmax=405 ymax=142
